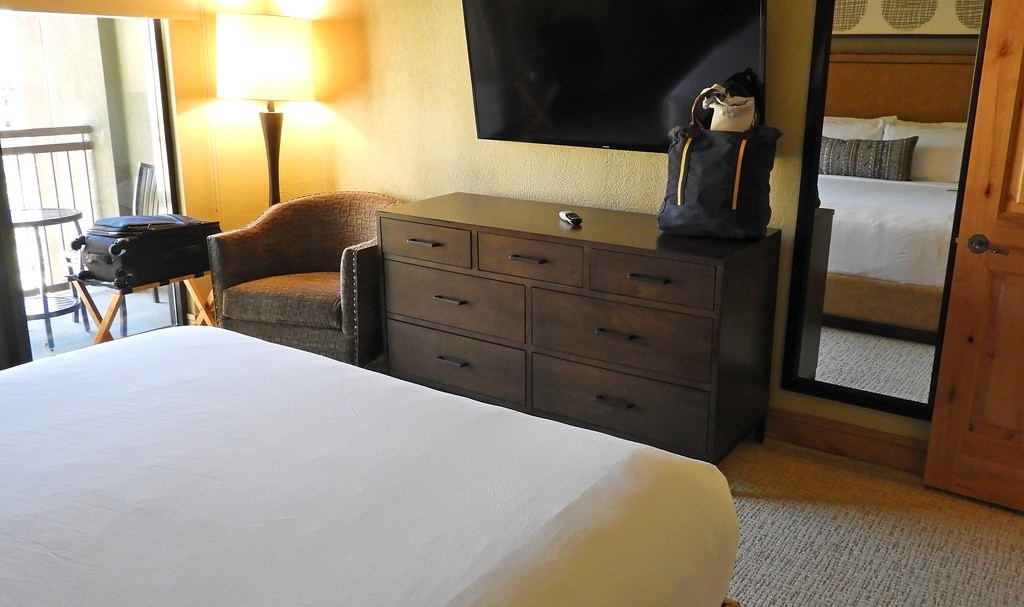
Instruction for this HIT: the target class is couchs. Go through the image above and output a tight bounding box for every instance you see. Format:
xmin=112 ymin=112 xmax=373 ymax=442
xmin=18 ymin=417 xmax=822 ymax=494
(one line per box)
xmin=206 ymin=191 xmax=412 ymax=369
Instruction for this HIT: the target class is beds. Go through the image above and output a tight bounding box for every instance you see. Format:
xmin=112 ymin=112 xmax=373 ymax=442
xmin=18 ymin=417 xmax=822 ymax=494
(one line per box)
xmin=0 ymin=326 xmax=738 ymax=607
xmin=818 ymin=174 xmax=958 ymax=344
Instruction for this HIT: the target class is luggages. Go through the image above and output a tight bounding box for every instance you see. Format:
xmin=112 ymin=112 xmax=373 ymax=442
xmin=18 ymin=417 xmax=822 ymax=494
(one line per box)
xmin=72 ymin=214 xmax=223 ymax=287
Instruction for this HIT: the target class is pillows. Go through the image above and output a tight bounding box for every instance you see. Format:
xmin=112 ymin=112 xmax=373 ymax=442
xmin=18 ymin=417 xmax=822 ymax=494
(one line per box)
xmin=823 ymin=115 xmax=899 ymax=140
xmin=880 ymin=118 xmax=968 ymax=183
xmin=815 ymin=136 xmax=919 ymax=182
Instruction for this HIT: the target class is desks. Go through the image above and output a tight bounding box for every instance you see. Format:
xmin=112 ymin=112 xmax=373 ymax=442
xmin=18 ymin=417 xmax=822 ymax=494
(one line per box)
xmin=9 ymin=207 xmax=91 ymax=351
xmin=63 ymin=270 xmax=217 ymax=345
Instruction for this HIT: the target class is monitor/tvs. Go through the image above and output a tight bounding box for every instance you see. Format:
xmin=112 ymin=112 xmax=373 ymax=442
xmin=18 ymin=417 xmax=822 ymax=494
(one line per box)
xmin=462 ymin=0 xmax=766 ymax=154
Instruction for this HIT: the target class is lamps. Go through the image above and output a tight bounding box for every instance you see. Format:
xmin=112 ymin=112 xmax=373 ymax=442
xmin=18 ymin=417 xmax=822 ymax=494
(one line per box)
xmin=215 ymin=11 xmax=317 ymax=207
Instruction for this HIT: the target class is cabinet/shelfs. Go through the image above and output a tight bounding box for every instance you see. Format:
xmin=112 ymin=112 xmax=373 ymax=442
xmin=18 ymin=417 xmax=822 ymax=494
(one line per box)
xmin=798 ymin=208 xmax=836 ymax=379
xmin=376 ymin=191 xmax=782 ymax=464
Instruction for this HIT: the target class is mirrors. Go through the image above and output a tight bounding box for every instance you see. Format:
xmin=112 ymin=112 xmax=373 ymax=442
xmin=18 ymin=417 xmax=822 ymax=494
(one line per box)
xmin=778 ymin=0 xmax=992 ymax=421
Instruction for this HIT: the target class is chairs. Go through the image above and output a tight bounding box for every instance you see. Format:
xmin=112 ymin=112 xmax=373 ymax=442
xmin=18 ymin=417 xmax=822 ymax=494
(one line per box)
xmin=61 ymin=161 xmax=160 ymax=336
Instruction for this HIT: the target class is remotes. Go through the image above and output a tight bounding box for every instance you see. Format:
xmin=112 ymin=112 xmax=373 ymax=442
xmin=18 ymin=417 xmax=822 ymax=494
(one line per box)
xmin=559 ymin=210 xmax=582 ymax=225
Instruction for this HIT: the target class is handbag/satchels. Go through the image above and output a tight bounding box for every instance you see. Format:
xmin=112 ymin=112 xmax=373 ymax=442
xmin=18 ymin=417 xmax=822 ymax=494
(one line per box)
xmin=656 ymin=85 xmax=783 ymax=241
